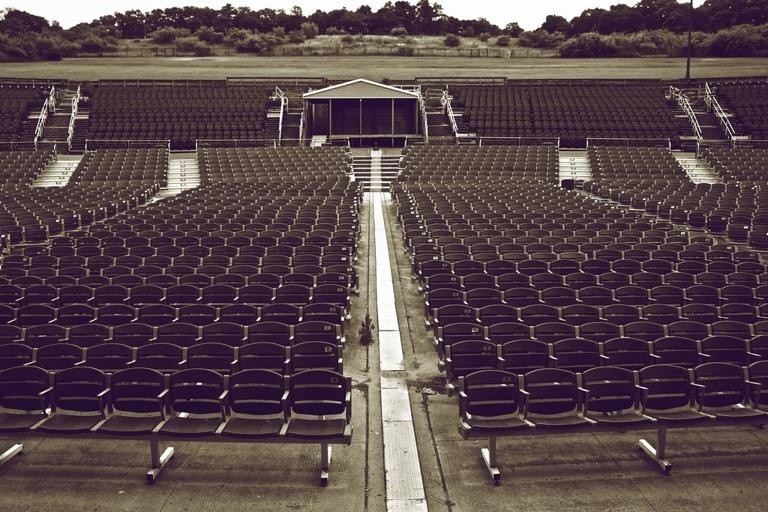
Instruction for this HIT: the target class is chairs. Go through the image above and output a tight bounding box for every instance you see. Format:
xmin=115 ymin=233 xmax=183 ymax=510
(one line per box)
xmin=1 ymin=72 xmax=365 ymax=490
xmin=382 ymin=73 xmax=765 ymax=485
